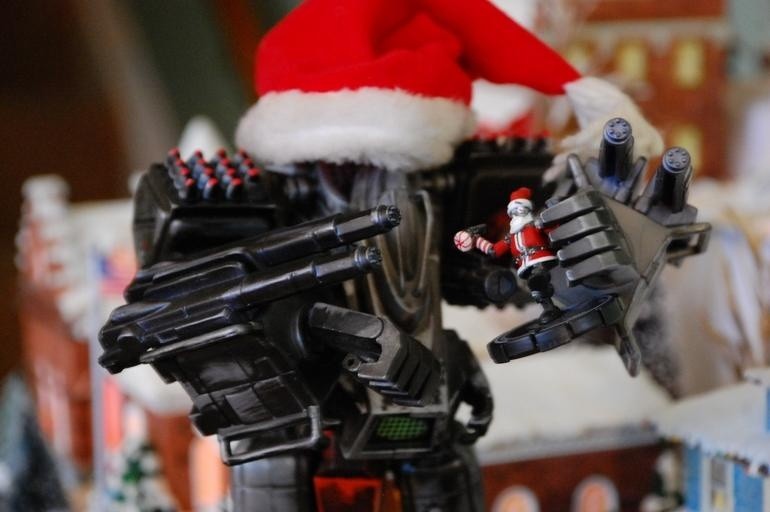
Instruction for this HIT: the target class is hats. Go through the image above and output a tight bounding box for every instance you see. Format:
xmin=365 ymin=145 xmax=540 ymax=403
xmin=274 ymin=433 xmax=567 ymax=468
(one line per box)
xmin=234 ymin=3 xmax=668 ymax=167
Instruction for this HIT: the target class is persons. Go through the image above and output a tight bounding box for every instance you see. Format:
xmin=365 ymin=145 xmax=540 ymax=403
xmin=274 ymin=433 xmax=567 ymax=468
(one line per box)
xmin=453 ymin=185 xmax=562 ymax=326
